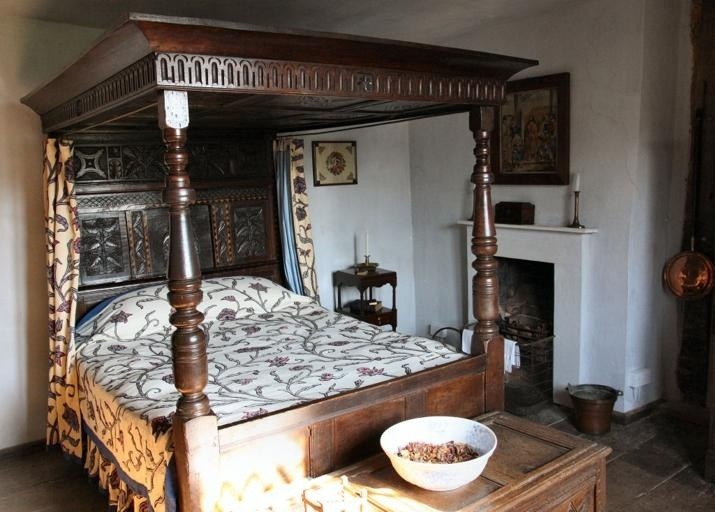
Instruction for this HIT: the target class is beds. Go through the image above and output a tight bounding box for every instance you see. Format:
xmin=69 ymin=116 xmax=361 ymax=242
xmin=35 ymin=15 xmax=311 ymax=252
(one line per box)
xmin=64 ymin=269 xmax=509 ymax=512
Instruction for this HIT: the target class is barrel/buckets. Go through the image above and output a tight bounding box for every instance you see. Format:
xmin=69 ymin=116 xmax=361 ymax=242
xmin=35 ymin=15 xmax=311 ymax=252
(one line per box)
xmin=564 ymin=380 xmax=623 ymax=436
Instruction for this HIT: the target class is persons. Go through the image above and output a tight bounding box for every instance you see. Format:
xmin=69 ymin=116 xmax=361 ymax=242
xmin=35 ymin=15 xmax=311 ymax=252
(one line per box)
xmin=502 ymin=112 xmax=556 ymax=171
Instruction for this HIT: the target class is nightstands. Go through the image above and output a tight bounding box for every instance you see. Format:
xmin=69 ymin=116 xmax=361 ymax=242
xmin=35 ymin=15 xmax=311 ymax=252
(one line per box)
xmin=333 ymin=269 xmax=398 ymax=333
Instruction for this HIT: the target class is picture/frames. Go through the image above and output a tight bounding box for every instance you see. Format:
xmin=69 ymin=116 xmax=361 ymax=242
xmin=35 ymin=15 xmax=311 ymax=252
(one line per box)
xmin=490 ymin=71 xmax=572 ymax=186
xmin=311 ymin=140 xmax=359 ymax=186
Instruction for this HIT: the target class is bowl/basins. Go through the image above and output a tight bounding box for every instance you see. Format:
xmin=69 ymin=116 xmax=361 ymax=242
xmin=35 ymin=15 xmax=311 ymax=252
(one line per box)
xmin=379 ymin=415 xmax=498 ymax=491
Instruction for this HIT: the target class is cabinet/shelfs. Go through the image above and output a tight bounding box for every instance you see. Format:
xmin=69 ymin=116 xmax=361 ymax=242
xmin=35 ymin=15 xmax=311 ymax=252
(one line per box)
xmin=510 ymin=460 xmax=615 ymax=512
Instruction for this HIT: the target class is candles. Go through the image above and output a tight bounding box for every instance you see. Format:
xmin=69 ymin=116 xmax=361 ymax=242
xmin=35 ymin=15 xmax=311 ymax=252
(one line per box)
xmin=573 ymin=169 xmax=582 ymax=191
xmin=364 ymin=232 xmax=370 ymax=254
